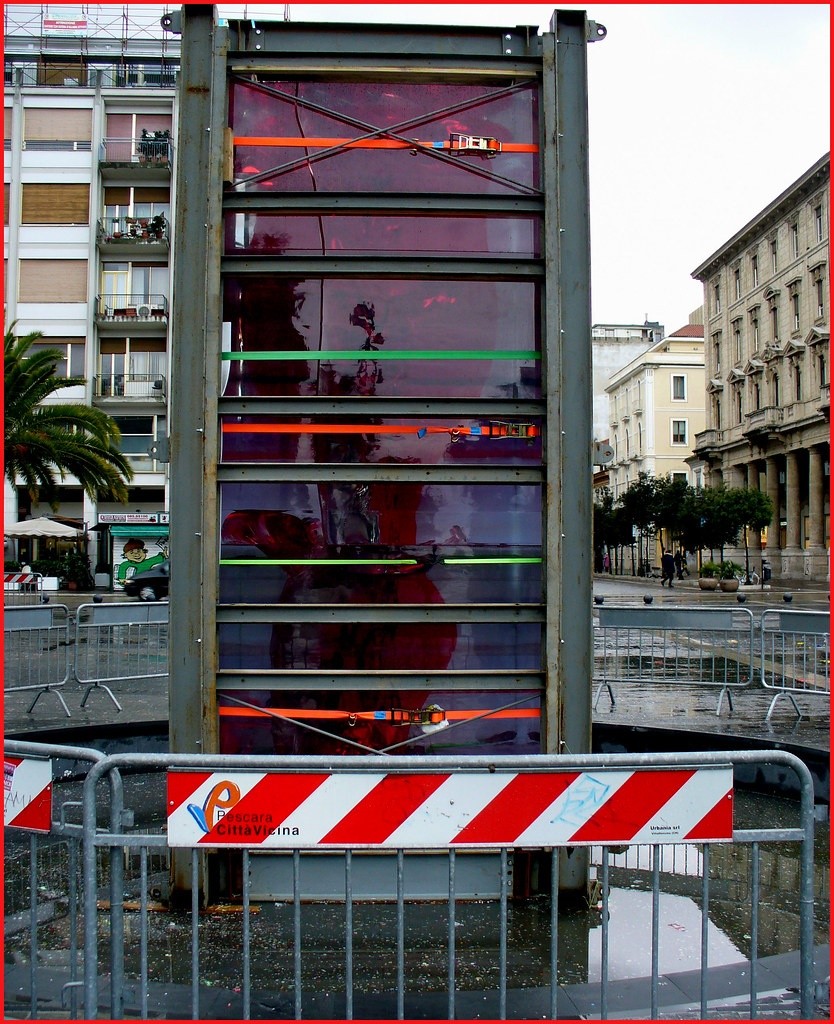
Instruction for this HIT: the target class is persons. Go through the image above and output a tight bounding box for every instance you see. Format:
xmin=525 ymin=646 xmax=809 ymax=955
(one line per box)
xmin=69 ymin=542 xmax=80 ymax=556
xmin=603 ymin=553 xmax=610 ymax=572
xmin=674 ymin=550 xmax=690 ymax=580
xmin=661 ymin=550 xmax=676 ymax=588
xmin=44 ymin=541 xmax=57 ymax=561
xmin=22 ymin=560 xmax=35 ymax=591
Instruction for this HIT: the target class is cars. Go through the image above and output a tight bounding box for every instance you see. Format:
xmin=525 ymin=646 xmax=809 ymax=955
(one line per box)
xmin=123 ymin=557 xmax=172 ymax=602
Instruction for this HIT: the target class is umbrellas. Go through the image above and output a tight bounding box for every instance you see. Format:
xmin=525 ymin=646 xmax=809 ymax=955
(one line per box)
xmin=4 ymin=517 xmax=84 ymax=541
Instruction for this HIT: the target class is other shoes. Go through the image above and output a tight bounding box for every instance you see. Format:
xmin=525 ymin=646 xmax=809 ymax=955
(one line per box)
xmin=669 ymin=585 xmax=674 ymax=587
xmin=661 ymin=580 xmax=665 ymax=586
